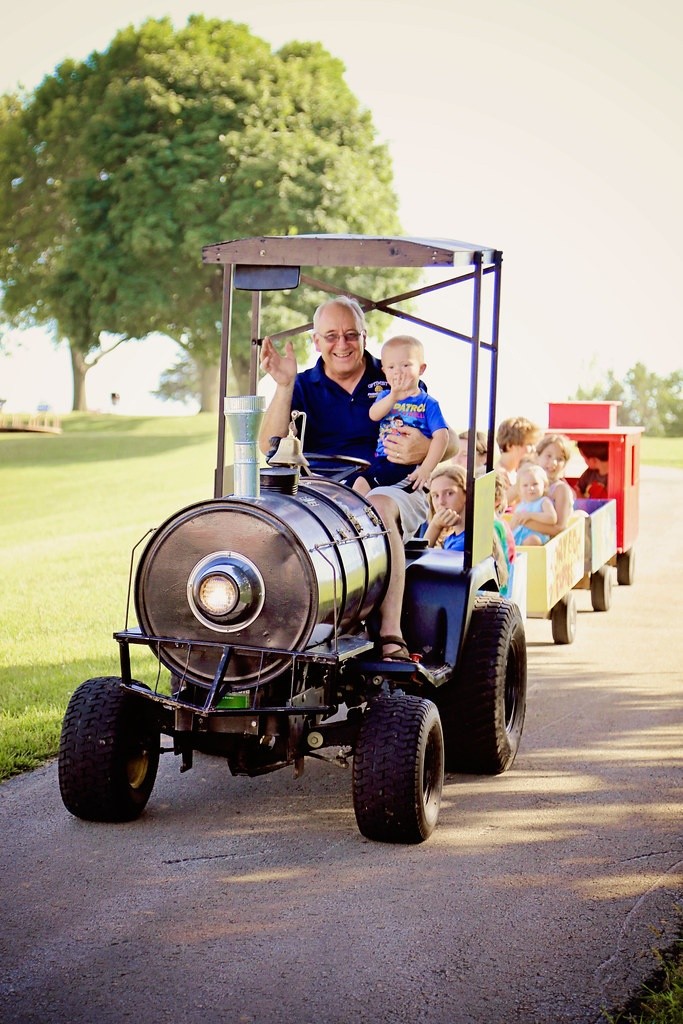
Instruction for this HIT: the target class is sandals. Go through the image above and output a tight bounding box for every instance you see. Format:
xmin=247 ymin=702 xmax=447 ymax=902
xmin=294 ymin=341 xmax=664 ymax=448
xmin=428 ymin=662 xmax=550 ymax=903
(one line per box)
xmin=376 ymin=635 xmax=410 ymax=661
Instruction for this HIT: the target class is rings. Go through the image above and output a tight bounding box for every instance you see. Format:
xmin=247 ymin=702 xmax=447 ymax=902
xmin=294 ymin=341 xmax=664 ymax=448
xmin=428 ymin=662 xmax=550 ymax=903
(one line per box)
xmin=395 ymin=451 xmax=400 ymax=459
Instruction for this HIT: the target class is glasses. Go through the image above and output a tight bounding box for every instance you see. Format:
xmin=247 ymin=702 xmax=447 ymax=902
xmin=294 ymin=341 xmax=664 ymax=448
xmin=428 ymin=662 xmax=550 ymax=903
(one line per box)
xmin=317 ymin=329 xmax=364 ymax=343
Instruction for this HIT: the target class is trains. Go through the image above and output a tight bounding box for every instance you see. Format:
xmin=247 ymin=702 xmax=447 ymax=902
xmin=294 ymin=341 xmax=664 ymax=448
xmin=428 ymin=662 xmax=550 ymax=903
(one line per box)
xmin=54 ymin=234 xmax=643 ymax=846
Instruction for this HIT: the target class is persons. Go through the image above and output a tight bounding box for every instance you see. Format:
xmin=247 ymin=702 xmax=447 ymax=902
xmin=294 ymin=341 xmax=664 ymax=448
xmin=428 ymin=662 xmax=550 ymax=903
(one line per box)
xmin=506 ymin=463 xmax=558 ymax=548
xmin=419 ymin=462 xmax=467 ymax=553
xmin=575 ymin=439 xmax=610 ymax=499
xmin=495 ymin=416 xmax=544 ymax=489
xmin=474 ymin=464 xmax=516 ymax=601
xmin=506 ymin=434 xmax=574 ymax=540
xmin=339 ymin=336 xmax=450 ymax=498
xmin=254 ymin=291 xmax=460 ymax=665
xmin=446 ymin=430 xmax=488 ymax=471
